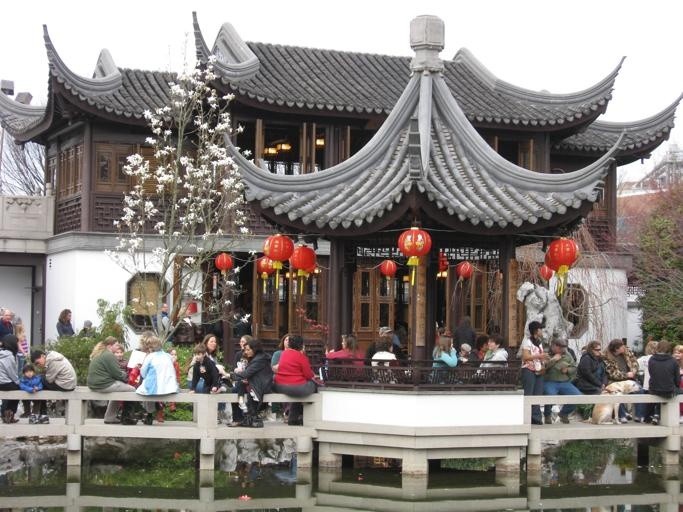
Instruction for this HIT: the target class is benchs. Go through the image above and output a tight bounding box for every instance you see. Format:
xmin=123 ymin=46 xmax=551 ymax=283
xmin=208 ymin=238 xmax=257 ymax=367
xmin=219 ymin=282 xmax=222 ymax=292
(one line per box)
xmin=0 ymin=466 xmax=683 ymax=512
xmin=1 ymin=386 xmax=314 ymax=428
xmin=525 ymin=391 xmax=683 ymax=428
xmin=325 ymin=358 xmax=520 ymax=392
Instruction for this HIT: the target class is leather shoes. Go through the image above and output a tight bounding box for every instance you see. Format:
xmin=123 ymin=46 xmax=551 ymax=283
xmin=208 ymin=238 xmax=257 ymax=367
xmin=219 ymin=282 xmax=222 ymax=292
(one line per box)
xmin=123 ymin=417 xmax=138 ymax=424
xmin=142 ymin=417 xmax=152 ymax=425
xmin=104 ymin=418 xmax=121 ymax=424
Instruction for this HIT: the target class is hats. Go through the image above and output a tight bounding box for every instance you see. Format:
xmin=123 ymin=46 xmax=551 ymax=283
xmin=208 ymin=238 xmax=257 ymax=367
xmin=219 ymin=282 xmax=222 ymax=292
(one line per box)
xmin=528 ymin=321 xmax=545 ymax=328
xmin=378 ymin=326 xmax=393 ymax=337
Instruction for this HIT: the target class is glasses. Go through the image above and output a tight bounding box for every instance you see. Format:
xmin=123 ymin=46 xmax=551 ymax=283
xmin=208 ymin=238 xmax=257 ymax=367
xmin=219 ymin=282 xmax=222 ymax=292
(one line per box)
xmin=593 ymin=348 xmax=602 ymax=351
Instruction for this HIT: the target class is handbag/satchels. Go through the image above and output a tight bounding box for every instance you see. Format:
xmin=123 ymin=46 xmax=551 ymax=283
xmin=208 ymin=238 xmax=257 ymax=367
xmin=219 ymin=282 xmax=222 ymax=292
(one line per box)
xmin=240 ymin=416 xmax=264 ymax=428
xmin=288 ymin=405 xmax=303 ymax=425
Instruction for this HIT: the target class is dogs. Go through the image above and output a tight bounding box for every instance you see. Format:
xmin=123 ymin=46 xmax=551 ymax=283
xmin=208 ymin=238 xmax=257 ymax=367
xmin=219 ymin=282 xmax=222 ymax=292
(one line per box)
xmin=582 ymin=379 xmax=642 ymax=425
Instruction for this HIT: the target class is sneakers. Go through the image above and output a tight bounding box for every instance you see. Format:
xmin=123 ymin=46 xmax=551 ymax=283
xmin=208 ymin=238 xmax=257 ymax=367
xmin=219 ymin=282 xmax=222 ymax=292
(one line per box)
xmin=545 ymin=416 xmax=552 ymax=424
xmin=27 ymin=414 xmax=42 ymax=424
xmin=227 ymin=421 xmax=241 ymax=426
xmin=558 ymin=413 xmax=569 ymax=423
xmin=38 ymin=415 xmax=50 ymax=424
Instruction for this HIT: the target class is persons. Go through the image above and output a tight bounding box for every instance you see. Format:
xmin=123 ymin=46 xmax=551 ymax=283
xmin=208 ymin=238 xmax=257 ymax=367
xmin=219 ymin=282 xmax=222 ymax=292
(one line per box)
xmin=187 ymin=334 xmax=272 ymax=428
xmin=87 ymin=332 xmax=180 ymax=425
xmin=153 ymin=304 xmax=251 ymax=345
xmin=79 ymin=321 xmax=94 ymax=337
xmin=1 ymin=308 xmax=28 ymax=376
xmin=520 ymin=321 xmax=683 ymax=425
xmin=270 ymin=333 xmax=322 ymax=425
xmin=234 ymin=462 xmax=262 ymax=489
xmin=1 ymin=334 xmax=77 ymax=424
xmin=57 ymin=309 xmax=74 ymax=337
xmin=325 ymin=314 xmax=510 ymax=384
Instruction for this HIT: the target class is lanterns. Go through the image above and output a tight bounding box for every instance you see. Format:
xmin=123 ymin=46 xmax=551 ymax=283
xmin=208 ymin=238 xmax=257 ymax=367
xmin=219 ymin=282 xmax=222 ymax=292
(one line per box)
xmin=257 ymin=234 xmax=318 ymax=295
xmin=540 ymin=236 xmax=579 ymax=296
xmin=381 ymin=260 xmax=396 ymax=290
xmin=215 ymin=252 xmax=233 ymax=275
xmin=439 ymin=253 xmax=447 ymax=272
xmin=456 ymin=261 xmax=473 ymax=288
xmin=398 ymin=227 xmax=432 ymax=285
